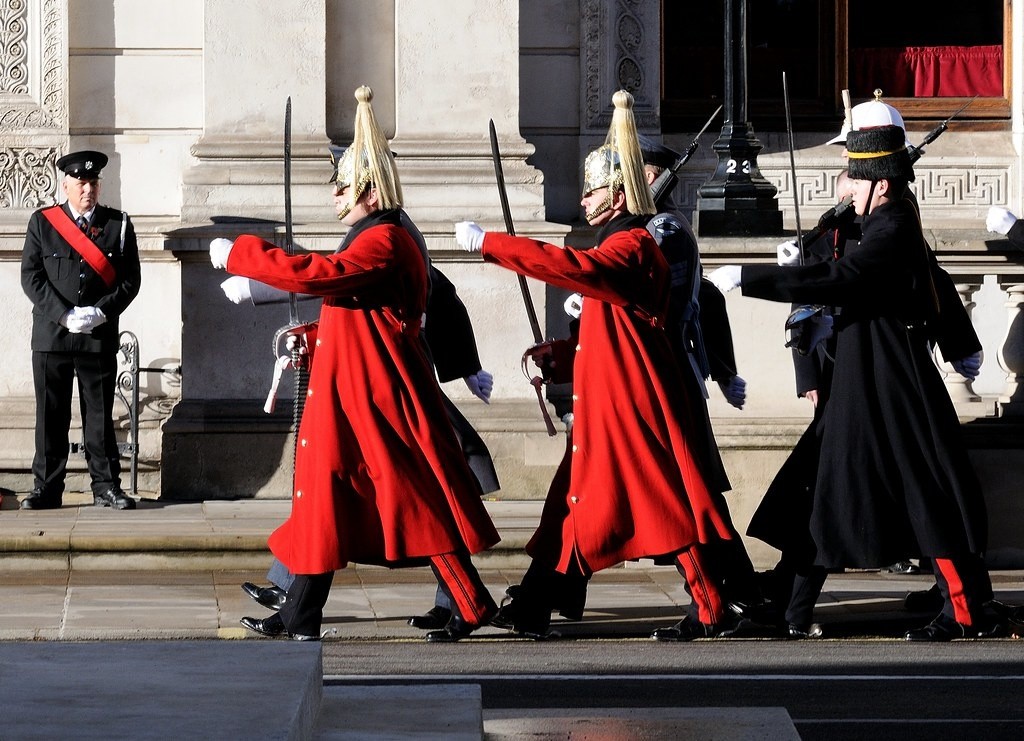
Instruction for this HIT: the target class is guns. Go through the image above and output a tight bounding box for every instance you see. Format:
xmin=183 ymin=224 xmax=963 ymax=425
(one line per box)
xmin=650 ymin=102 xmax=725 ymax=207
xmin=794 ymin=91 xmax=983 ymax=250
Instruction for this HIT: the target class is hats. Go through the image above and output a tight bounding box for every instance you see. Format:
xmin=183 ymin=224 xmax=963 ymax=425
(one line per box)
xmin=635 ymin=130 xmax=681 ymax=171
xmin=56 ymin=150 xmax=108 ymax=180
xmin=328 ymin=146 xmax=397 ymax=184
xmin=841 ymin=89 xmax=915 ymax=183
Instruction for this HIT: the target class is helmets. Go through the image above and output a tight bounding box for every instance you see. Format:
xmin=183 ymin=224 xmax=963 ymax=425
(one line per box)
xmin=826 ymin=90 xmax=926 ymax=156
xmin=582 ymin=90 xmax=657 ymax=222
xmin=334 ymin=84 xmax=404 ymax=220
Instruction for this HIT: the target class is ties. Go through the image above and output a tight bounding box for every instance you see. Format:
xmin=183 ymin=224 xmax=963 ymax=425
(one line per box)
xmin=78 ymin=216 xmax=88 ymax=235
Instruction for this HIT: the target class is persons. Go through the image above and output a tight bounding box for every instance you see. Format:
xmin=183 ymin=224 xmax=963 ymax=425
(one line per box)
xmin=20 ymin=151 xmax=142 ymax=509
xmin=209 ymin=85 xmax=500 ymax=642
xmin=455 ymin=89 xmax=735 ymax=643
xmin=492 ymin=132 xmax=769 ymax=634
xmin=701 ymin=89 xmax=1024 ymax=637
xmin=220 ymin=146 xmax=501 ymax=630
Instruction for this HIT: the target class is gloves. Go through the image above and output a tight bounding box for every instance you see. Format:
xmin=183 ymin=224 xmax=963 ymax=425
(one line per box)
xmin=463 ymin=370 xmax=493 ymax=405
xmin=563 ymin=293 xmax=584 ymax=321
xmin=454 ymin=221 xmax=486 ymax=253
xmin=796 ymin=315 xmax=833 ymax=359
xmin=776 ymin=239 xmax=800 ymax=267
xmin=986 ymin=206 xmax=1017 ymax=237
xmin=59 ymin=305 xmax=107 ymax=334
xmin=950 ymin=352 xmax=980 ymax=381
xmin=220 ymin=275 xmax=251 ymax=304
xmin=532 ymin=345 xmax=556 ymax=369
xmin=285 ymin=332 xmax=308 ymax=355
xmin=718 ymin=375 xmax=746 ymax=410
xmin=707 ymin=265 xmax=742 ymax=292
xmin=209 ymin=238 xmax=235 ymax=269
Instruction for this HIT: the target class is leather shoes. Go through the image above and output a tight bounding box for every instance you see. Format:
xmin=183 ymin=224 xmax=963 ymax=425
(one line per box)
xmin=94 ymin=486 xmax=136 ymax=510
xmin=240 ymin=560 xmax=1024 ymax=646
xmin=21 ymin=487 xmax=62 ymax=509
